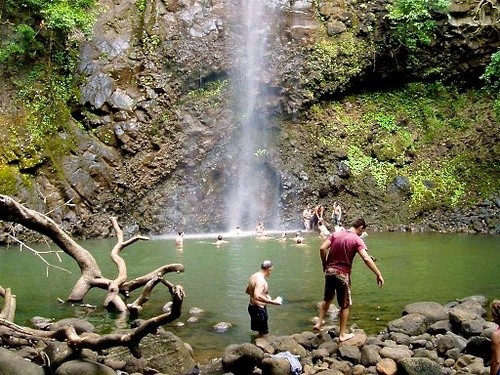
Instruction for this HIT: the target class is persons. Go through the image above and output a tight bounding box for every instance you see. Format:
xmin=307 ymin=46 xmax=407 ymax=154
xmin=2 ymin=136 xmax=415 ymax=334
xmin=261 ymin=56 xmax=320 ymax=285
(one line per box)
xmin=313 ymin=219 xmax=384 ymax=342
xmin=245 ymin=260 xmax=282 ymax=338
xmin=488 ymin=299 xmax=500 ymax=375
xmin=175 ymin=201 xmax=347 ymax=252
